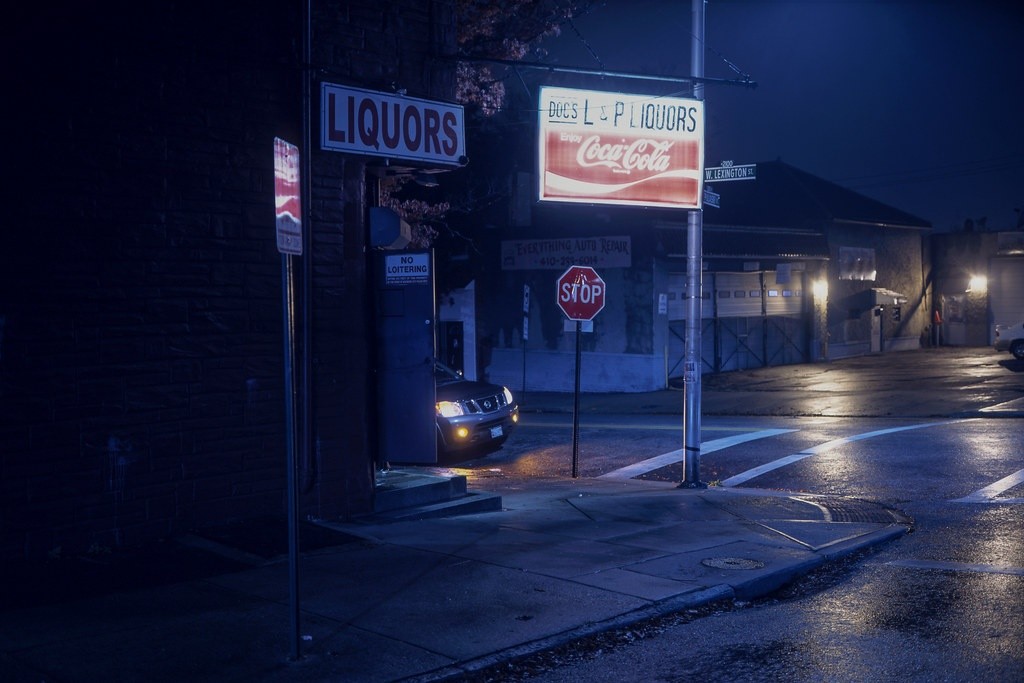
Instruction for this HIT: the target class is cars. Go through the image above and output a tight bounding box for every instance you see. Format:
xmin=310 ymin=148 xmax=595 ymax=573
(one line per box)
xmin=434 ymin=359 xmax=520 ymax=461
xmin=994 ymin=321 xmax=1024 ymax=360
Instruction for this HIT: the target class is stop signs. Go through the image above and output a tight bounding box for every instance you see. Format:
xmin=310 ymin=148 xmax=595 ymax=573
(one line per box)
xmin=558 ymin=266 xmax=605 ymax=321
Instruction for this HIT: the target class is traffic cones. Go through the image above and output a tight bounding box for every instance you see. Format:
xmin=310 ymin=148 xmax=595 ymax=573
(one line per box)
xmin=934 ymin=310 xmax=942 ymax=323
